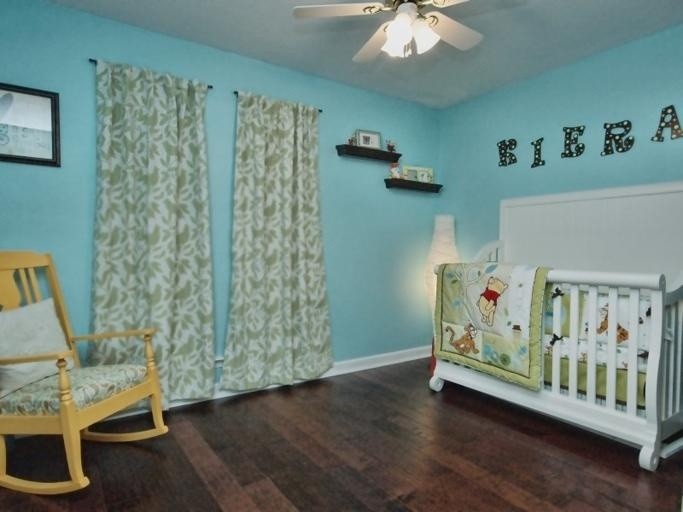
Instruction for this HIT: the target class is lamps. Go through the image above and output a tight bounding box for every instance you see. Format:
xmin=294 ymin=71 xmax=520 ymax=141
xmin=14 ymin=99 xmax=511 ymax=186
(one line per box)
xmin=380 ymin=3 xmax=440 ymax=58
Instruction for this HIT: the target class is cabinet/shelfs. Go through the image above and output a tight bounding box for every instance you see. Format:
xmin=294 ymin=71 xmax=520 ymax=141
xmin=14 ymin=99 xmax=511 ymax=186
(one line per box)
xmin=336 ymin=144 xmax=443 ymax=193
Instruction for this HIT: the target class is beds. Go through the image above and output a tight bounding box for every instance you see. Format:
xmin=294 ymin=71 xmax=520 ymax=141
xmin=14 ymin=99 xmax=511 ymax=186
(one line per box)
xmin=429 ymin=182 xmax=683 ymax=472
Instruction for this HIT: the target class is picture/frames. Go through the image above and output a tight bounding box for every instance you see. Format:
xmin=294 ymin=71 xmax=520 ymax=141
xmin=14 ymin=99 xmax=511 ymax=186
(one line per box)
xmin=0 ymin=82 xmax=61 ymax=167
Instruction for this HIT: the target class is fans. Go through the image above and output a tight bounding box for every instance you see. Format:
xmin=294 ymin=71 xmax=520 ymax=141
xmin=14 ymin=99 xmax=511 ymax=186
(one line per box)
xmin=293 ymin=0 xmax=484 ymax=63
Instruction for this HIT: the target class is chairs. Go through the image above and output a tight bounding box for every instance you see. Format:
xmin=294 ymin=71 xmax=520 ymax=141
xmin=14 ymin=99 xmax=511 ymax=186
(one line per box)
xmin=0 ymin=250 xmax=169 ymax=496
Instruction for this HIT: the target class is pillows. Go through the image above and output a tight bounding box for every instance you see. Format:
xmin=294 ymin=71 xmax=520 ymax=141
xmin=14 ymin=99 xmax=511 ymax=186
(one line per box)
xmin=0 ymin=298 xmax=76 ymax=399
